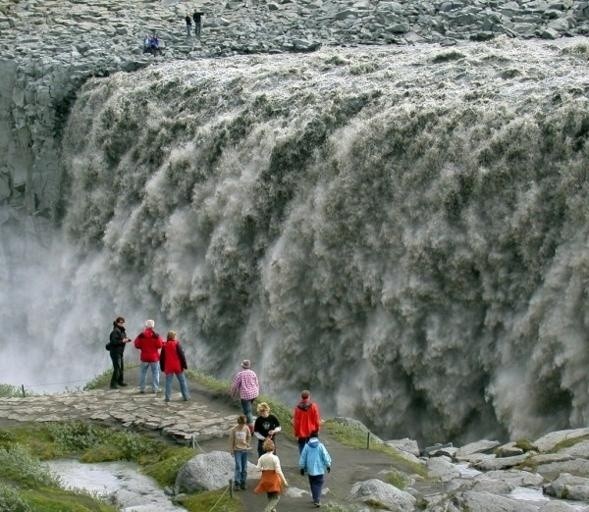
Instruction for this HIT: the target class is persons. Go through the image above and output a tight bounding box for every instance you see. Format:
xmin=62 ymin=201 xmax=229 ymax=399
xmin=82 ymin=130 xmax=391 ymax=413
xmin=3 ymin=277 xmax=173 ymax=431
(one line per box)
xmin=186 ymin=12 xmax=193 ymax=39
xmin=252 ymin=402 xmax=283 ymax=460
xmin=106 ymin=316 xmax=131 ymax=390
xmin=150 ymin=33 xmax=166 ymax=57
xmin=192 ymin=8 xmax=205 ymax=36
xmin=254 ymin=436 xmax=289 ymax=512
xmin=158 ymin=329 xmax=192 ymax=402
xmin=133 ymin=318 xmax=162 ymax=393
xmin=142 ymin=34 xmax=151 ymax=53
xmin=229 ymin=359 xmax=259 ymax=434
xmin=229 ymin=415 xmax=253 ymax=493
xmin=294 ymin=389 xmax=321 ymax=477
xmin=298 ymin=427 xmax=331 ymax=508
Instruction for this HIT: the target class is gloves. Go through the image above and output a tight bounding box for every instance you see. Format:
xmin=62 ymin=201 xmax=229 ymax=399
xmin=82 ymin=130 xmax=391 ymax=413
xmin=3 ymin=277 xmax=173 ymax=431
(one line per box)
xmin=326 ymin=466 xmax=331 ymax=473
xmin=300 ymin=468 xmax=304 ymax=475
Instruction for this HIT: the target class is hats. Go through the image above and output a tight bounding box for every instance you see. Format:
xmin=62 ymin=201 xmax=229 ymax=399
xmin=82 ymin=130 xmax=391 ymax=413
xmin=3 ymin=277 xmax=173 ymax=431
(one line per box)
xmin=145 ymin=319 xmax=155 ymax=328
xmin=241 ymin=359 xmax=251 ymax=369
